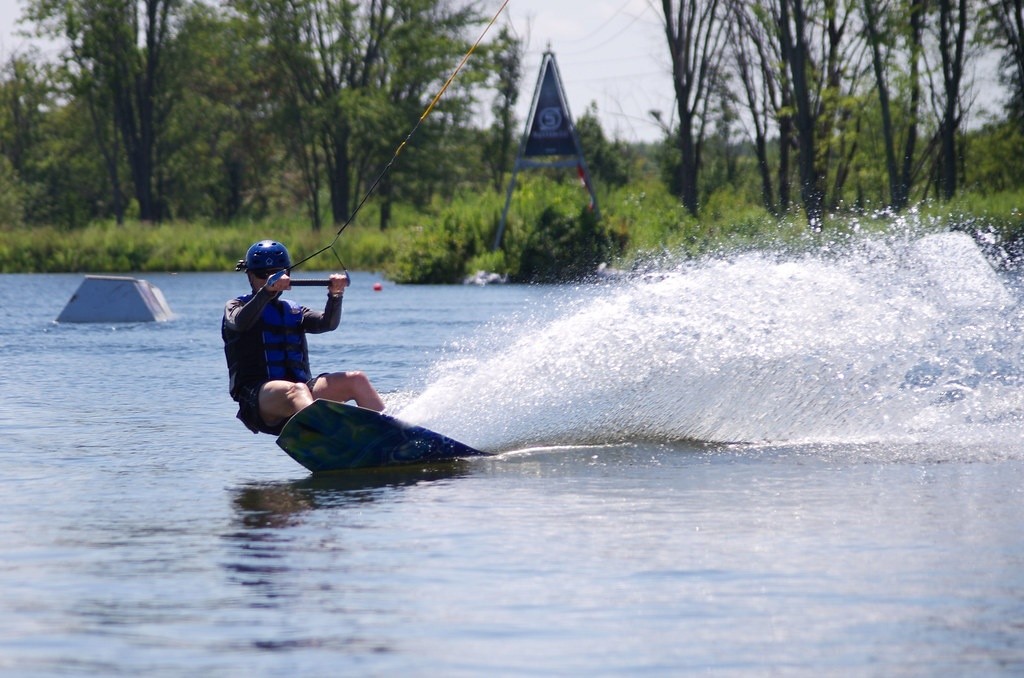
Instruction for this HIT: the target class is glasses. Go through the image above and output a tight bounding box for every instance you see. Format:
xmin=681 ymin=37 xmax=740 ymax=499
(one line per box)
xmin=252 ymin=268 xmax=291 ymax=280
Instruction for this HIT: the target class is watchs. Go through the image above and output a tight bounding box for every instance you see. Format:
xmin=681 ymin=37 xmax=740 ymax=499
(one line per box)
xmin=327 ymin=287 xmax=345 ymax=298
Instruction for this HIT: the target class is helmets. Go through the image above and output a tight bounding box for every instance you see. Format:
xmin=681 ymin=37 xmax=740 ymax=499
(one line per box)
xmin=245 ymin=240 xmax=290 ymax=270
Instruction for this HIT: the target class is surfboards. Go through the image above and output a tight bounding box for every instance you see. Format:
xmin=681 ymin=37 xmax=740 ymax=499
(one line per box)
xmin=277 ymin=397 xmax=498 ymax=472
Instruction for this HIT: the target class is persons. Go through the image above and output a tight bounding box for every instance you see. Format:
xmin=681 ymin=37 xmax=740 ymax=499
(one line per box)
xmin=221 ymin=240 xmax=385 ymax=437
